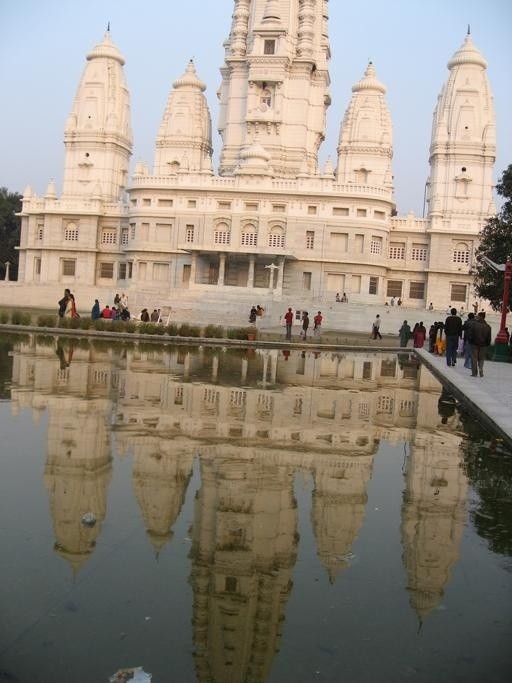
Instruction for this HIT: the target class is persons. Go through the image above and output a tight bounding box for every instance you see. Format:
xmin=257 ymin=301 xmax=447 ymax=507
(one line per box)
xmin=436 ymin=387 xmax=454 ymax=423
xmin=63 ymin=293 xmax=76 ymax=318
xmin=284 ymin=307 xmax=294 ymax=335
xmin=398 ymin=296 xmax=402 ymax=305
xmin=444 ymin=307 xmax=461 ymax=366
xmin=92 ymin=298 xmax=100 ymax=319
xmin=398 ymin=315 xmax=446 ymax=354
xmin=447 ymin=305 xmax=452 ymax=314
xmin=300 ymin=350 xmax=306 ymax=358
xmin=414 ymin=355 xmax=421 ymax=369
xmin=282 ymin=348 xmax=290 ymax=361
xmin=56 ymin=339 xmax=70 ymax=369
xmin=390 ymin=295 xmax=396 ymax=305
xmin=460 ymin=313 xmax=477 ymax=368
xmin=370 ymin=313 xmax=384 ymax=339
xmin=336 ymin=293 xmax=340 ymax=301
xmin=249 ymin=305 xmax=265 ymax=321
xmin=103 ymin=293 xmax=132 ymax=319
xmin=151 ymin=309 xmax=159 ymax=320
xmin=429 ymin=302 xmax=433 ymax=309
xmin=469 ymin=311 xmax=492 ymax=377
xmin=341 ymin=292 xmax=349 ymax=301
xmin=298 ymin=311 xmax=310 ymax=339
xmin=396 ymin=353 xmax=409 ymax=370
xmin=312 ymin=310 xmax=322 ymax=332
xmin=57 ymin=288 xmax=70 ymax=316
xmin=312 ymin=350 xmax=321 ymax=359
xmin=140 ymin=308 xmax=151 ymax=320
